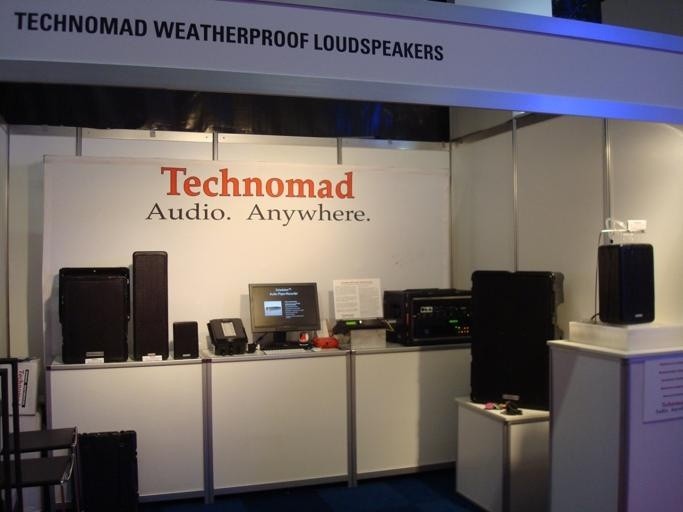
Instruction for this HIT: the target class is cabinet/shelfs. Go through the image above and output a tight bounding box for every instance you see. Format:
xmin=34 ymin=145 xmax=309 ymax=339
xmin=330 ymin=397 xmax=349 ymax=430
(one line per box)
xmin=454 ymin=395 xmax=549 ymax=512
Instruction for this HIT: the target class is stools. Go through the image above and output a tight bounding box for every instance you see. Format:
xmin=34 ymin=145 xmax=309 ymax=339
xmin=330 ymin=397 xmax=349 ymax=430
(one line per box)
xmin=0 ymin=426 xmax=81 ymax=512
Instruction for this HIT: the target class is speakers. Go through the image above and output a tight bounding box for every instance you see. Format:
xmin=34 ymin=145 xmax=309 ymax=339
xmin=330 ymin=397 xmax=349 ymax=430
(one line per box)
xmin=598 ymin=244 xmax=655 ymax=323
xmin=59 ymin=267 xmax=130 ymax=363
xmin=173 ymin=322 xmax=198 ymax=359
xmin=469 ymin=271 xmax=564 ymax=412
xmin=133 ymin=251 xmax=169 ymax=360
xmin=78 ymin=431 xmax=139 ymax=512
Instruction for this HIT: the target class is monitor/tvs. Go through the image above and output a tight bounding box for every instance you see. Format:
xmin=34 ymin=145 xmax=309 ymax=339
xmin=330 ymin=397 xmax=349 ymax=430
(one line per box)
xmin=249 ymin=283 xmax=321 ymax=350
